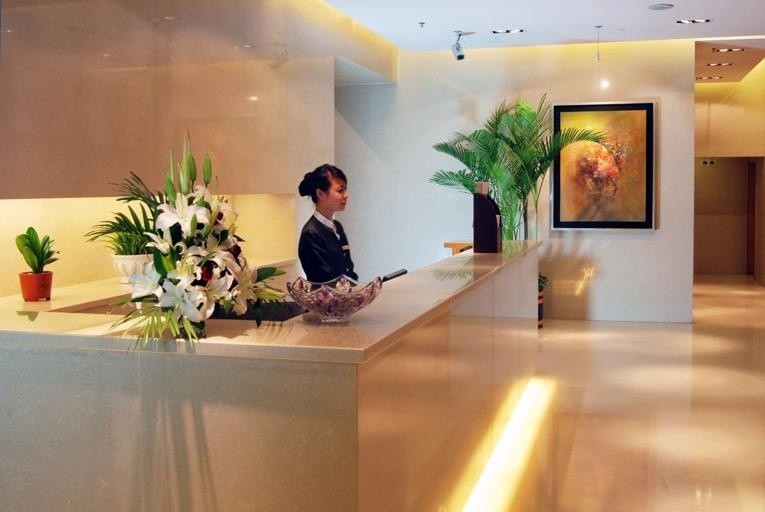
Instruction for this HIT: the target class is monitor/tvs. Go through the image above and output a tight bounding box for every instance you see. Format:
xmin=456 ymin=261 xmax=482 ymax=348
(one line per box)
xmin=383 ymin=268 xmax=408 ymax=281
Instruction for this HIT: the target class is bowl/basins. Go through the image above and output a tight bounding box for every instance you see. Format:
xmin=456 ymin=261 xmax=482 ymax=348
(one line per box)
xmin=288 ymin=276 xmax=383 ymax=324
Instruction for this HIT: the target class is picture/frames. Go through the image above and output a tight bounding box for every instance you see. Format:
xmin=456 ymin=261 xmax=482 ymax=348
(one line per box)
xmin=548 ymin=102 xmax=656 ymax=233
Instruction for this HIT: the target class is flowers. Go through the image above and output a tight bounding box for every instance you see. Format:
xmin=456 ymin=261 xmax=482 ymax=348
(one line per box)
xmin=82 ymin=128 xmax=291 ymax=345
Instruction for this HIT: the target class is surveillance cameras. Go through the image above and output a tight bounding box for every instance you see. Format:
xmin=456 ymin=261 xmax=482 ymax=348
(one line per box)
xmin=452 ymin=45 xmax=465 ymax=60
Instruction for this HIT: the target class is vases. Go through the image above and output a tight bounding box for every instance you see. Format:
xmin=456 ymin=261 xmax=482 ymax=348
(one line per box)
xmin=176 ymin=296 xmax=209 ymax=339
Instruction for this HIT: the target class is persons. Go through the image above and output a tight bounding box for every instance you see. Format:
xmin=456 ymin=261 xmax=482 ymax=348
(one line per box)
xmin=298 ymin=163 xmax=359 ymax=314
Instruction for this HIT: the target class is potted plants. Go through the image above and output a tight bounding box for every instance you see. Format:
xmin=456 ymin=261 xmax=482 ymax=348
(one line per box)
xmin=429 ymin=90 xmax=612 ymax=330
xmin=97 ymin=231 xmax=154 ymax=286
xmin=14 ymin=226 xmax=59 ymax=301
